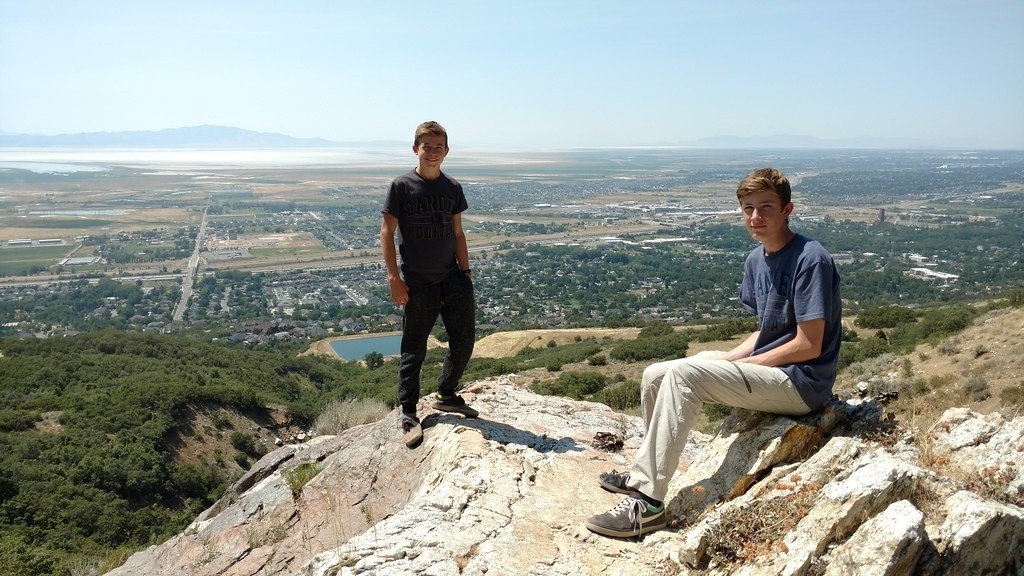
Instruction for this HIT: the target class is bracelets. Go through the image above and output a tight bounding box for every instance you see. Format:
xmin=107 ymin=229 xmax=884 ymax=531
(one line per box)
xmin=462 ymin=269 xmax=471 ymax=273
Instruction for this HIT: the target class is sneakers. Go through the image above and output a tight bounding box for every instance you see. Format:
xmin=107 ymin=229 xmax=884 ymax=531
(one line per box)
xmin=432 ymin=393 xmax=479 ymax=417
xmin=586 ymin=491 xmax=666 ymax=537
xmin=598 ymin=469 xmax=631 ymax=494
xmin=402 ymin=416 xmax=422 ymax=445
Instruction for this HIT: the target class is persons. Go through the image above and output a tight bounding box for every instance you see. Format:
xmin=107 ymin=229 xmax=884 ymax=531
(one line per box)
xmin=585 ymin=169 xmax=842 ymax=537
xmin=379 ymin=120 xmax=478 ymax=446
